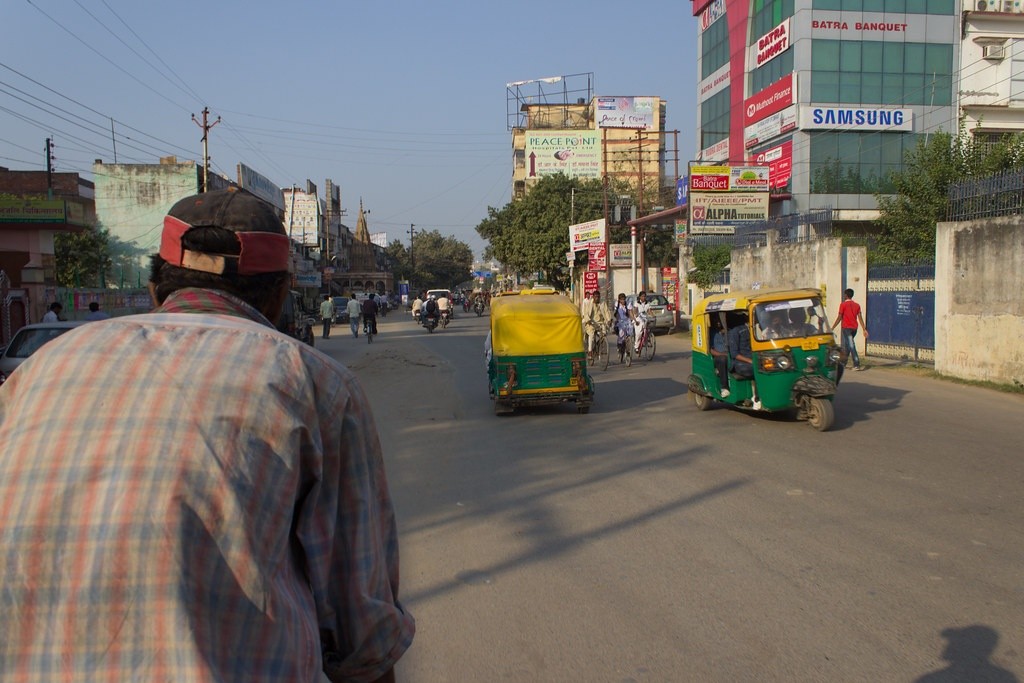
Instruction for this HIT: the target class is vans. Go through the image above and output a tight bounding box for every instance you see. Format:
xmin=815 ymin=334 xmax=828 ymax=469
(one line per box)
xmin=426 ymin=289 xmax=454 ymax=320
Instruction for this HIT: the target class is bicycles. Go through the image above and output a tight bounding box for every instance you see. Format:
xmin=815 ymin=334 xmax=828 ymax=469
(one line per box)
xmin=363 ymin=313 xmax=378 ymax=344
xmin=583 ymin=313 xmax=656 ymax=373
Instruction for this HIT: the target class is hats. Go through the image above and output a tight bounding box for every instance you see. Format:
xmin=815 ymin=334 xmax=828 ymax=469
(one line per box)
xmin=477 ymin=292 xmax=481 ymax=295
xmin=160 ymin=186 xmax=289 ymax=275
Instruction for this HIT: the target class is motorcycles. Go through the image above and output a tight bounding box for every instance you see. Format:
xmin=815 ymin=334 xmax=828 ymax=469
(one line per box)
xmin=686 ymin=285 xmax=841 ymax=432
xmin=486 ymin=286 xmax=595 ymax=418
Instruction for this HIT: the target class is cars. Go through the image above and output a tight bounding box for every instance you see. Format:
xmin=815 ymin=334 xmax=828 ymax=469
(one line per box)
xmin=0 ymin=320 xmax=90 ymax=386
xmin=329 ymin=297 xmax=351 ymax=324
xmin=354 ymin=292 xmax=398 ymax=317
xmin=625 ymin=292 xmax=674 ymax=337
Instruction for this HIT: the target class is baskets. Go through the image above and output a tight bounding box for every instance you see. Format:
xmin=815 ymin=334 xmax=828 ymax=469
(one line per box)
xmin=642 ymin=316 xmax=656 ymax=328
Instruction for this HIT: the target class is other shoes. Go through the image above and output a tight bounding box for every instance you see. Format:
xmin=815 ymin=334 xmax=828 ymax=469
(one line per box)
xmin=617 ymin=347 xmax=624 ymax=353
xmin=721 ymin=389 xmax=730 ymax=397
xmin=852 ymin=365 xmax=859 ymax=371
xmin=635 ymin=348 xmax=639 ymax=354
xmin=587 ymin=352 xmax=592 ymax=359
xmin=648 ymin=341 xmax=652 ymax=347
xmin=364 ymin=327 xmax=366 ymax=331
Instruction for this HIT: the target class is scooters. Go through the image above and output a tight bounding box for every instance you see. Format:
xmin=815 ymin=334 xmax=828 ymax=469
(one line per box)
xmin=453 ymin=295 xmax=490 ymax=317
xmin=412 ymin=305 xmax=451 ymax=333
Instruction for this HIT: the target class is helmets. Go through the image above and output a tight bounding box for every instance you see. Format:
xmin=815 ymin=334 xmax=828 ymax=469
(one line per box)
xmin=432 ymin=295 xmax=436 ymax=299
xmin=427 ymin=295 xmax=432 ymax=299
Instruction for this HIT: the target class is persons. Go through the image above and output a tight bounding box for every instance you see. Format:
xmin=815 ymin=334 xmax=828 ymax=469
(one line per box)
xmin=381 ymin=292 xmax=387 ymax=318
xmin=581 ymin=291 xmax=612 ymax=360
xmin=709 ymin=308 xmax=823 ymax=410
xmin=633 ymin=292 xmax=654 ymax=353
xmin=0 ymin=187 xmax=417 ymax=683
xmin=615 ymin=293 xmax=640 ymax=354
xmin=84 ymin=302 xmax=109 ymax=321
xmin=831 ymin=288 xmax=868 ymax=371
xmin=43 ymin=302 xmax=62 ymax=322
xmin=319 ymin=295 xmax=334 ymax=339
xmin=347 ymin=294 xmax=360 ymax=339
xmin=363 ymin=294 xmax=377 ymax=335
xmin=411 ymin=290 xmax=490 ymax=328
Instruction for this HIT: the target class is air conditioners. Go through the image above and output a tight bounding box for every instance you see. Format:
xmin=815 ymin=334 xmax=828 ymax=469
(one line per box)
xmin=983 ymin=45 xmax=1005 ymax=59
xmin=1000 ymin=0 xmax=1024 ymax=13
xmin=974 ymin=0 xmax=1000 ymax=12
